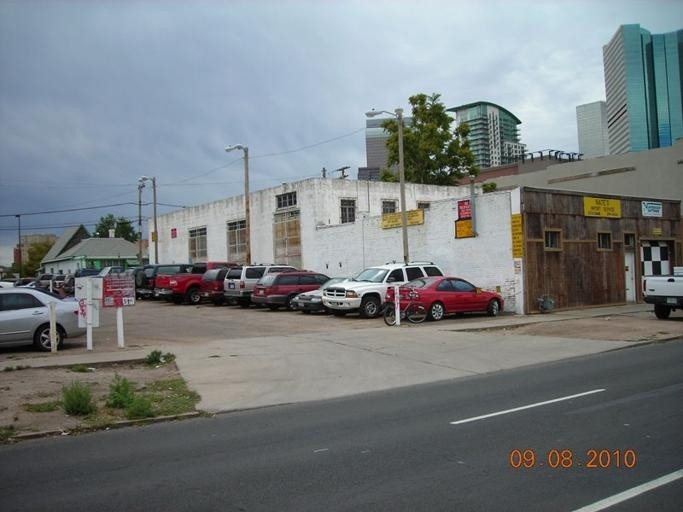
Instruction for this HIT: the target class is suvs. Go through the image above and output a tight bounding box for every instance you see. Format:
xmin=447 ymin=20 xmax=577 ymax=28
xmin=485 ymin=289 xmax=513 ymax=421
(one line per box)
xmin=222 ymin=263 xmax=300 ymax=308
xmin=250 ymin=269 xmax=331 ymax=312
xmin=322 ymin=262 xmax=446 ymax=318
xmin=99 ymin=261 xmax=239 ymax=306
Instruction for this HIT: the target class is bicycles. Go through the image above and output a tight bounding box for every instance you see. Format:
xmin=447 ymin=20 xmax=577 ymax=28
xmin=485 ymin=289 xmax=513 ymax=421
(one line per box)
xmin=381 ymin=286 xmax=427 ymax=326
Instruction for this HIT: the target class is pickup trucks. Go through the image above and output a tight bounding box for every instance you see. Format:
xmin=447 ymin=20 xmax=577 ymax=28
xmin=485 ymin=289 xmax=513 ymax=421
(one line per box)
xmin=642 ymin=272 xmax=683 ymax=319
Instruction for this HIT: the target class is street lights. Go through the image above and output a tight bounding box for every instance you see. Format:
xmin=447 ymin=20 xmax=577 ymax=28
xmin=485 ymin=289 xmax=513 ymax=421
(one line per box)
xmin=138 ymin=176 xmax=158 ymax=265
xmin=364 ymin=108 xmax=409 ymax=264
xmin=224 ymin=143 xmax=251 ymax=266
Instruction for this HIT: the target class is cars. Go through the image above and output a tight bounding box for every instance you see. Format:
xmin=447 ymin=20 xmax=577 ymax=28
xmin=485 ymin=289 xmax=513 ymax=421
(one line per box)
xmin=384 ymin=276 xmax=504 ymax=321
xmin=1 ymin=267 xmax=98 ymax=351
xmin=290 ymin=277 xmax=354 ymax=315
